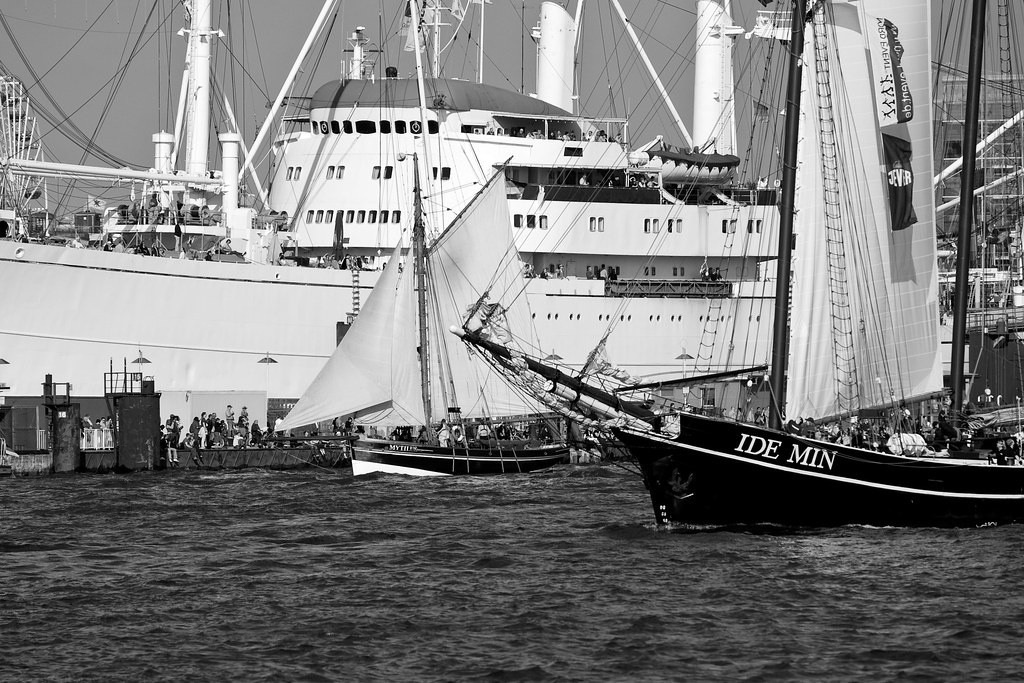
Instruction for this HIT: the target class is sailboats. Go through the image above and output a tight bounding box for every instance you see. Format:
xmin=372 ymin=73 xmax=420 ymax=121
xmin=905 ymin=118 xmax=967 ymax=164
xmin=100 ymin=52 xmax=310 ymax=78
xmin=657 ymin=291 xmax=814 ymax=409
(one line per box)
xmin=0 ymin=1 xmax=1024 ymax=531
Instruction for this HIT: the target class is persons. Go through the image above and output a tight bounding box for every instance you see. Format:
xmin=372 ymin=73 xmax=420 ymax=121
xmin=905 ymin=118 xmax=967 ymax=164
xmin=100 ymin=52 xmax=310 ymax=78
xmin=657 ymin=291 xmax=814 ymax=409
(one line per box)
xmin=84 ymin=384 xmax=1023 ymax=465
xmin=939 ymin=301 xmax=947 ymax=324
xmin=20 ymin=125 xmax=769 ymax=280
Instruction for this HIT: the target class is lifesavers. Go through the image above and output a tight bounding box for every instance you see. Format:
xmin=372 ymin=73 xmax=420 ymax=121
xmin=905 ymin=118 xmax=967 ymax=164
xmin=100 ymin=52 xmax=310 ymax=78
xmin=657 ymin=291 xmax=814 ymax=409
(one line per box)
xmin=452 ymin=426 xmax=464 ymax=442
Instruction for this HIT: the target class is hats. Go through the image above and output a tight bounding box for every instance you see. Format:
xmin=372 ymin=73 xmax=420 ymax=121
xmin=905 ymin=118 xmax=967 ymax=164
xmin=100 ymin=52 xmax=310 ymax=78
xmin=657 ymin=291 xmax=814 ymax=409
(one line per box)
xmin=805 ymin=417 xmax=814 ymax=423
